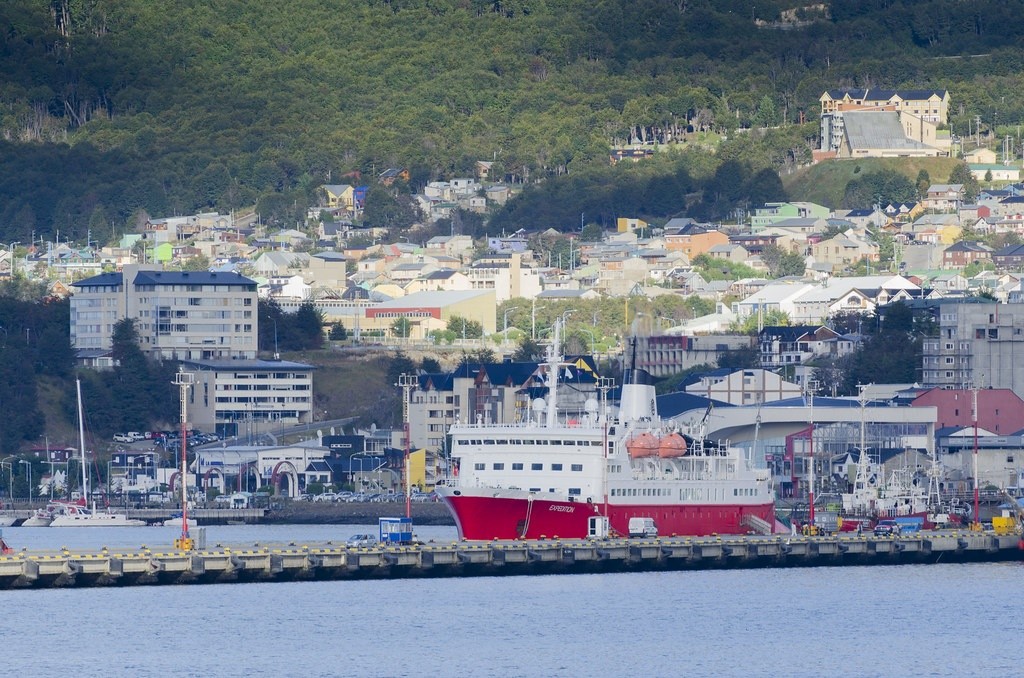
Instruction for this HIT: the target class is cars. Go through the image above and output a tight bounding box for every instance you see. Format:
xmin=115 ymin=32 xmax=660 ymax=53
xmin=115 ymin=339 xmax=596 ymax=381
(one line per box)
xmin=161 ymin=430 xmax=223 ymax=452
xmin=292 ymin=492 xmax=440 ymax=503
xmin=145 ymin=432 xmax=152 ymax=439
xmin=151 ymin=432 xmax=167 ymax=438
xmin=346 ymin=533 xmax=376 ymax=550
xmin=153 ymin=439 xmax=169 ymax=446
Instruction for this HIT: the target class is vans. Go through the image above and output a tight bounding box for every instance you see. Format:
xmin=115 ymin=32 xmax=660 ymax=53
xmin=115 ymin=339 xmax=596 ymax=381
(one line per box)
xmin=629 ymin=516 xmax=658 ymax=539
xmin=112 ymin=432 xmax=134 ymax=444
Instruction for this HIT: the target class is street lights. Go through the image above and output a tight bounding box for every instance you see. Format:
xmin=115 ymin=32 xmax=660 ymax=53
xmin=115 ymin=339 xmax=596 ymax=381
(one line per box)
xmin=503 ymin=306 xmax=519 ymax=349
xmin=10 ymin=240 xmax=22 ymax=282
xmin=375 ymin=457 xmax=381 ymax=496
xmin=0 ymin=326 xmax=8 ymax=347
xmin=268 ymin=315 xmax=278 ymax=356
xmin=19 ymin=461 xmax=32 ymax=501
xmin=39 ymin=434 xmax=51 ymax=462
xmin=352 ymin=457 xmax=362 ymax=505
xmin=0 ymin=461 xmax=13 ymax=502
xmin=563 ymin=309 xmax=579 ymax=360
xmin=594 ymin=309 xmax=603 ymax=329
xmin=26 ymin=328 xmax=30 ymax=346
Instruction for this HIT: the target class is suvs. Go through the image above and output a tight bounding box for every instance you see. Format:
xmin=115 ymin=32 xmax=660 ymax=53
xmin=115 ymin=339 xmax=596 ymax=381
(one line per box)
xmin=127 ymin=432 xmax=145 ymax=441
xmin=874 ymin=520 xmax=901 ymax=537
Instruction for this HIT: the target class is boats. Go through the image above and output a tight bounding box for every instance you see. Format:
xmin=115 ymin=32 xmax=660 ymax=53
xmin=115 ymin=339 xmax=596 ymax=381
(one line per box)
xmin=164 ymin=518 xmax=198 ymax=526
xmin=21 ymin=502 xmax=86 ymax=527
xmin=803 ymin=382 xmax=1022 ymax=531
xmin=434 ymin=319 xmax=778 ymax=543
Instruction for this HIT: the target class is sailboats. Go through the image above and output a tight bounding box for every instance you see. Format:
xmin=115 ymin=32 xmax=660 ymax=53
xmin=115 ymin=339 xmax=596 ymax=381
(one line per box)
xmin=51 ymin=378 xmax=149 ymax=527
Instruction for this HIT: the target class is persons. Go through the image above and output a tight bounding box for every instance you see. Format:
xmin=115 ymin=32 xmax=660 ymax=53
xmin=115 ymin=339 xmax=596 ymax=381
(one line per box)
xmin=790 ymin=520 xmax=798 ymax=537
xmin=856 ymin=521 xmax=863 ymax=538
